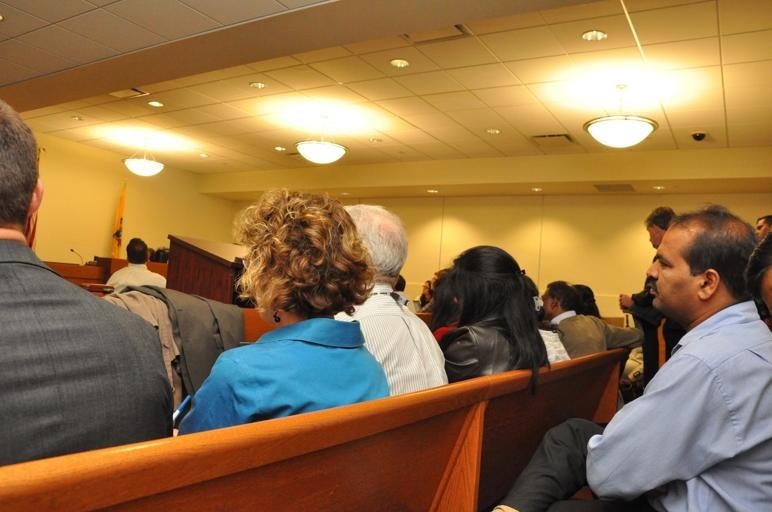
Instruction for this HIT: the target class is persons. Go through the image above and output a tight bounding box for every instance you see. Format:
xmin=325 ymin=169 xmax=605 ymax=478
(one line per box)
xmin=493 ymin=201 xmax=770 ymax=512
xmin=178 ymin=188 xmax=544 ymax=436
xmin=100 ymin=236 xmax=167 ymax=296
xmin=0 ymin=98 xmax=173 ymax=467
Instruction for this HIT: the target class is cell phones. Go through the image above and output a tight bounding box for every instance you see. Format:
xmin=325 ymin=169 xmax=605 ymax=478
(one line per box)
xmin=172 ymin=395 xmax=191 ymax=428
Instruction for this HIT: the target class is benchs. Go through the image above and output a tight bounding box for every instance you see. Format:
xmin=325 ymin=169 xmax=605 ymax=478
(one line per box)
xmin=0 ymin=343 xmax=627 ymax=511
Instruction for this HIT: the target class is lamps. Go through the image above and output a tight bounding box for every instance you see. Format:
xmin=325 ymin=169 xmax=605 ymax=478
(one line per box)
xmin=294 ymin=138 xmax=350 ymax=168
xmin=122 ymin=155 xmax=167 ymax=177
xmin=582 ymin=111 xmax=660 ymax=151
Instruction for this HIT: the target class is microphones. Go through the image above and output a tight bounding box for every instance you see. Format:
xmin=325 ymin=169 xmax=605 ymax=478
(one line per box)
xmin=70 ymin=248 xmax=83 ymax=264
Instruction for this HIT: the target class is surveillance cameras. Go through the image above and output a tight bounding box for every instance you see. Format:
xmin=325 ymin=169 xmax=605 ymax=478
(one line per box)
xmin=691 ymin=130 xmax=707 ymax=141
